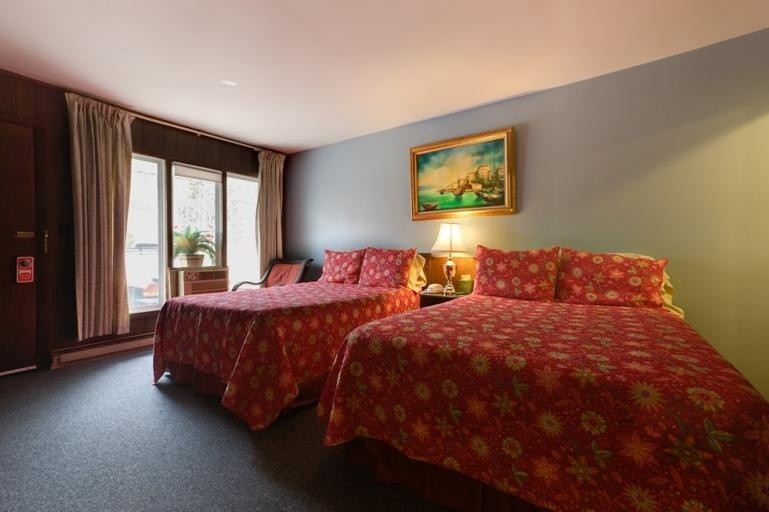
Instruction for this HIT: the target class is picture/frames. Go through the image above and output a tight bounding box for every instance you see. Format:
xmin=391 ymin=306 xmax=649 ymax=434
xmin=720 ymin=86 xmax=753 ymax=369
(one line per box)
xmin=409 ymin=125 xmax=517 ymax=221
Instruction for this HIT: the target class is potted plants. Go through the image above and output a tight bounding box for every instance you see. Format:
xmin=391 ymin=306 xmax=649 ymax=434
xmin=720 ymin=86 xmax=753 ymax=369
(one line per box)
xmin=173 ymin=224 xmax=217 ymax=267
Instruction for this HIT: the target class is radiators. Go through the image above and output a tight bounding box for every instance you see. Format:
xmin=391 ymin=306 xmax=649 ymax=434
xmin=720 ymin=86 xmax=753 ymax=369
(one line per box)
xmin=166 ymin=265 xmax=230 ymax=299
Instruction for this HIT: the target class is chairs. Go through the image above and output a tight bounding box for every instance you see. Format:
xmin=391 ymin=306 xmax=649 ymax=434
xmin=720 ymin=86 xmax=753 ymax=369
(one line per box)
xmin=232 ymin=258 xmax=314 ymax=291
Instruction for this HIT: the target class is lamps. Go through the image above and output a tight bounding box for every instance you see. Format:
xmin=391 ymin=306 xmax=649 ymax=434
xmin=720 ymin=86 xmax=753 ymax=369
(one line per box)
xmin=430 ymin=223 xmax=466 ymax=294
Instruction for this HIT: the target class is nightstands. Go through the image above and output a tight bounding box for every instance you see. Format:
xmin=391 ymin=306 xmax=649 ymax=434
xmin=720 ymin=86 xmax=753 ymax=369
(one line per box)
xmin=419 ymin=291 xmax=465 ymax=308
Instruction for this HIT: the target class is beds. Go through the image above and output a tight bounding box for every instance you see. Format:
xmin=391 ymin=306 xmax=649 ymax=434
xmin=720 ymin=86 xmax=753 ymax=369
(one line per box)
xmin=315 ymin=293 xmax=769 ymax=512
xmin=153 ymin=253 xmax=433 ymax=431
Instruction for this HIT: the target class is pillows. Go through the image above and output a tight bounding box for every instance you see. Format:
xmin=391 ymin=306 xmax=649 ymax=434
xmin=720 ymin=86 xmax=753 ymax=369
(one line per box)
xmin=472 ymin=244 xmax=674 ymax=308
xmin=317 ymin=246 xmax=428 ymax=293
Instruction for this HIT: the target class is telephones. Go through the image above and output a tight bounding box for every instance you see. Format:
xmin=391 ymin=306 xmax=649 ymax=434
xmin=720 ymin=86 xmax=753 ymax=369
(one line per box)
xmin=427 ymin=283 xmax=443 ymax=293
